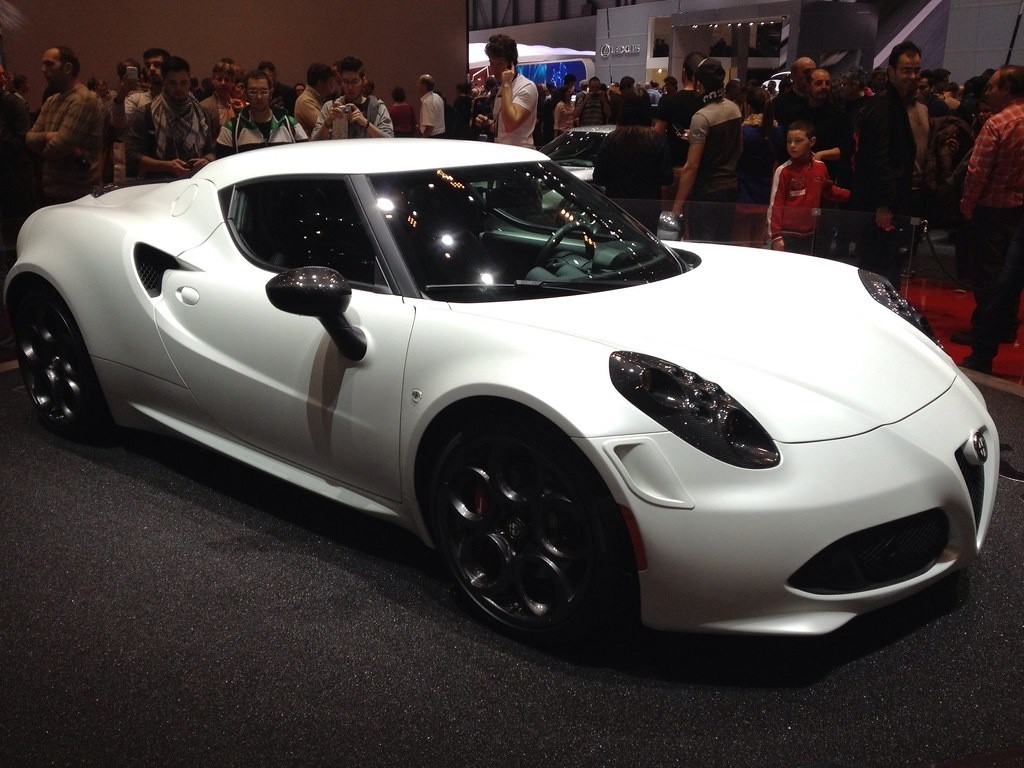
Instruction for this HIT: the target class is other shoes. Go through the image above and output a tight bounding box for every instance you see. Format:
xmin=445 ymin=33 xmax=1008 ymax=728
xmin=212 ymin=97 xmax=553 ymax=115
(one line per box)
xmin=959 ymin=354 xmax=993 ymax=370
xmin=900 ymin=267 xmax=913 ymax=278
xmin=951 ymin=325 xmax=1015 ymax=344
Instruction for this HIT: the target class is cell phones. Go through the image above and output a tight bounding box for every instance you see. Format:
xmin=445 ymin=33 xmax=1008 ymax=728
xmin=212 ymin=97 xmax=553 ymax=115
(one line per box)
xmin=125 ymin=67 xmax=138 ymax=79
xmin=672 ymin=122 xmax=686 ymax=136
xmin=507 ymin=57 xmax=518 ymax=70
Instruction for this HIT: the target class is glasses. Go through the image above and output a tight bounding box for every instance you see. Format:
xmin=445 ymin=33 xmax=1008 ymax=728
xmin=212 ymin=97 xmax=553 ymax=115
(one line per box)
xmin=942 ymin=79 xmax=949 ymax=82
xmin=840 ymin=82 xmax=849 ymax=87
xmin=248 ymin=89 xmax=270 ymax=96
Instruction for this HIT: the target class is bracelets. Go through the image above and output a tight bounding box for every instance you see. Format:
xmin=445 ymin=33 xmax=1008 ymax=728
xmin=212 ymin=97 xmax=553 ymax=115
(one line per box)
xmin=362 ymin=119 xmax=369 ymax=129
xmin=324 ymin=119 xmax=332 ymax=128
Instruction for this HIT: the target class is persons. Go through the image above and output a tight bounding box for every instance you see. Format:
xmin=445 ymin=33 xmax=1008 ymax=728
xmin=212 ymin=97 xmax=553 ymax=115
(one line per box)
xmin=0 ymin=33 xmax=1024 ymax=377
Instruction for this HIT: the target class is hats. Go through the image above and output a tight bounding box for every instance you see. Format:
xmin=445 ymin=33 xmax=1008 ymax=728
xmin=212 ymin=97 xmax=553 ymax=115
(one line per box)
xmin=578 ymin=80 xmax=589 ymax=87
xmin=780 ymin=79 xmax=793 ymax=91
xmin=694 ymin=58 xmax=726 ymax=88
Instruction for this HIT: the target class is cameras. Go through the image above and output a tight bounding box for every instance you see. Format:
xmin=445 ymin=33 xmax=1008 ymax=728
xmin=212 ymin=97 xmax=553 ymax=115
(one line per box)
xmin=338 ymin=104 xmax=351 ymax=115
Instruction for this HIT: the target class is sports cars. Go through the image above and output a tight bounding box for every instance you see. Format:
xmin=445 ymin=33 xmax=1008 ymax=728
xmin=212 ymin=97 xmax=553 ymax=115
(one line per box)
xmin=1 ymin=138 xmax=999 ymax=643
xmin=543 ymin=127 xmax=616 ymax=183
